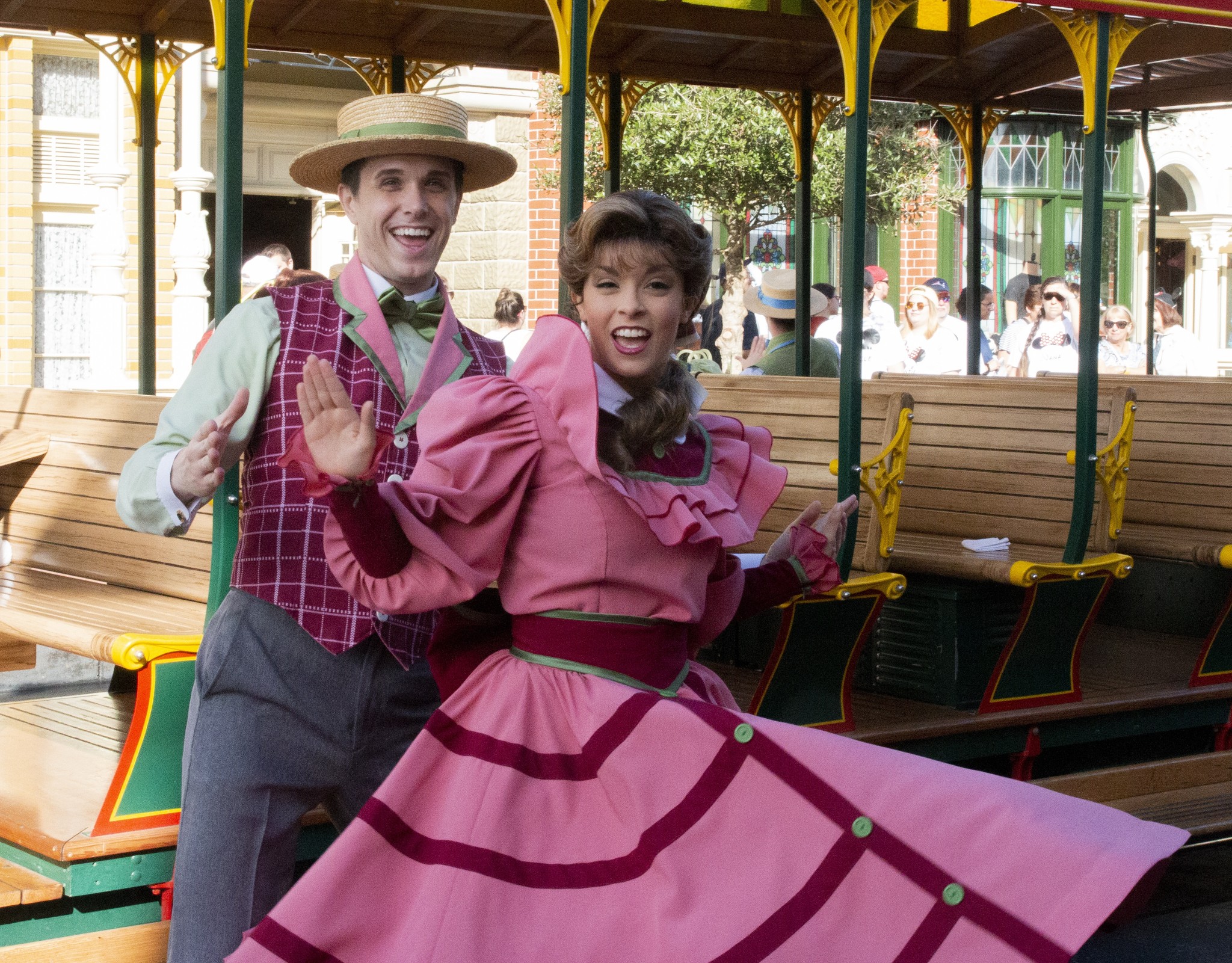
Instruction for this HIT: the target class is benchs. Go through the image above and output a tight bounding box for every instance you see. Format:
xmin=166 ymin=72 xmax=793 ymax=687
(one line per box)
xmin=872 ymin=370 xmax=1136 ymax=717
xmin=0 ymin=378 xmax=246 ymax=828
xmin=1036 ymin=367 xmax=1232 ymax=688
xmin=688 ymin=367 xmax=915 ymax=732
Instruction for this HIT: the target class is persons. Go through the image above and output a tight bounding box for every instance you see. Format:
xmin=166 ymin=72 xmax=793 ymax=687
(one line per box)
xmin=291 ymin=189 xmax=864 ymax=963
xmin=116 ymin=92 xmax=848 ymax=963
xmin=439 ymin=274 xmax=456 ymax=307
xmin=481 ymin=285 xmax=536 ymax=364
xmin=704 ymin=247 xmax=1208 ymax=380
xmin=258 ymin=243 xmax=294 ymax=273
xmin=188 ymin=252 xmax=282 ymax=371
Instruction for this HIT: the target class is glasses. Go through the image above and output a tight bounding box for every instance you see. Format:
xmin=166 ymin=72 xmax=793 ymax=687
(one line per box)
xmin=980 ymin=302 xmax=995 ymax=311
xmin=830 ymin=296 xmax=842 ymax=303
xmin=906 ymin=301 xmax=929 ymax=310
xmin=516 ymin=306 xmax=528 ymax=316
xmin=878 ymin=280 xmax=888 ymax=283
xmin=1104 ymin=320 xmax=1131 ymax=329
xmin=937 ymin=296 xmax=951 ymax=302
xmin=740 ymin=273 xmax=752 ymax=281
xmin=1041 ymin=293 xmax=1067 ymax=302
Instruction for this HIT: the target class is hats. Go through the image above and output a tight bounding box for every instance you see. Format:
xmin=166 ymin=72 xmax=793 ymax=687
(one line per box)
xmin=743 ymin=269 xmax=827 ymax=320
xmin=907 ymin=286 xmax=939 ymax=307
xmin=1154 ymin=292 xmax=1176 ymax=309
xmin=289 ymin=93 xmax=518 ymax=194
xmin=923 ymin=277 xmax=950 ymax=292
xmin=719 ymin=258 xmax=752 ymax=280
xmin=864 ymin=266 xmax=887 ymax=284
xmin=241 ymin=255 xmax=280 ymax=287
xmin=841 ymin=269 xmax=874 ymax=288
